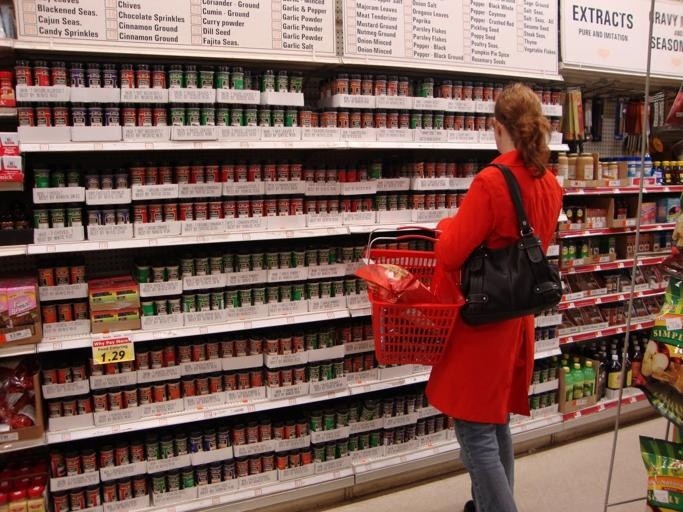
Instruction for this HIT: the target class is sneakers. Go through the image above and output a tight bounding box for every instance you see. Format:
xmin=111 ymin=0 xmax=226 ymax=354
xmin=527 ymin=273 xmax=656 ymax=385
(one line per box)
xmin=463 ymin=500 xmax=478 ymax=512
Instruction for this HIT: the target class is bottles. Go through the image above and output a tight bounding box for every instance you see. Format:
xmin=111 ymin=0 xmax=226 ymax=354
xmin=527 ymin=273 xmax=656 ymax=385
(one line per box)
xmin=559 ymin=153 xmax=683 ymax=188
xmin=554 ymin=333 xmax=649 ymax=400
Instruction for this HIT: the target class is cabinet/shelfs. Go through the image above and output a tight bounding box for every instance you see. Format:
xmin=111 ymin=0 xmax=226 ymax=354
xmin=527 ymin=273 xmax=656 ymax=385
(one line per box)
xmin=0 ymin=0 xmax=683 ymax=512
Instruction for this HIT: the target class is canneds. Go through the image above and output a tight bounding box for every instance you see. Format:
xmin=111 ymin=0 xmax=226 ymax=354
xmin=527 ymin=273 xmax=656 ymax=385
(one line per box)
xmin=15 ymin=59 xmax=562 ymax=512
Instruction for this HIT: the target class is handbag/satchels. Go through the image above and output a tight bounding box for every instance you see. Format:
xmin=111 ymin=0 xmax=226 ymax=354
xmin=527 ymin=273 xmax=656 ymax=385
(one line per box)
xmin=458 ymin=162 xmax=563 ymax=328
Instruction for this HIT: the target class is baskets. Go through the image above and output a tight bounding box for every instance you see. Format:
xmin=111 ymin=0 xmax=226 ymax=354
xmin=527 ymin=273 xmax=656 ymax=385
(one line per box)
xmin=363 ymin=225 xmax=467 ymax=366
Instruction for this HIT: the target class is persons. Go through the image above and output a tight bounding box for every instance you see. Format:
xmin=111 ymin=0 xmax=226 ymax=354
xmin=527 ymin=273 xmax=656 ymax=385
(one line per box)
xmin=422 ymin=81 xmax=563 ymax=511
